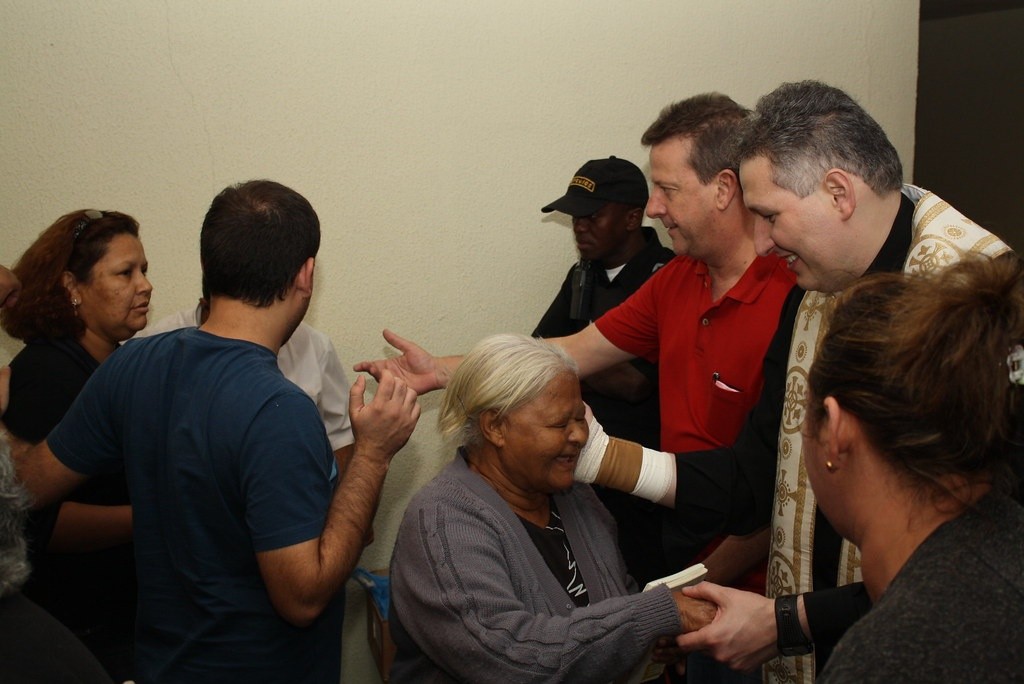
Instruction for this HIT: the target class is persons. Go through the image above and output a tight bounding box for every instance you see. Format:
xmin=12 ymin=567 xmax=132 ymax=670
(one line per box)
xmin=352 ymin=94 xmax=805 ymax=683
xmin=568 ymin=82 xmax=1013 ymax=684
xmin=529 ymin=153 xmax=675 ymax=588
xmin=803 ymin=245 xmax=1023 ymax=684
xmin=0 ymin=178 xmax=425 ymax=684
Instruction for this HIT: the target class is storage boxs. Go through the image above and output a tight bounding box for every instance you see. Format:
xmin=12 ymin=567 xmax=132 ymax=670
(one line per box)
xmin=353 ymin=568 xmax=397 ymax=682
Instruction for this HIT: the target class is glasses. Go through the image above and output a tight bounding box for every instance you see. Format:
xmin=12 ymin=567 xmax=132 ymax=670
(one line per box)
xmin=72 ymin=208 xmax=104 ymax=247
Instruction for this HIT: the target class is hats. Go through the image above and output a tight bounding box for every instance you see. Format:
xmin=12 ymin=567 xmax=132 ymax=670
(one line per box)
xmin=541 ymin=157 xmax=649 ymax=217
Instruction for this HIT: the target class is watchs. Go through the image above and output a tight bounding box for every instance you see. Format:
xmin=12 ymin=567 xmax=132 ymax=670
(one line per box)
xmin=774 ymin=594 xmax=815 ymax=657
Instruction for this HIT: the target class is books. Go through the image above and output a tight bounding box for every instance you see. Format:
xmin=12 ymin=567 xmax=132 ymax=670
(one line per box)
xmin=641 ymin=563 xmax=709 ymax=592
